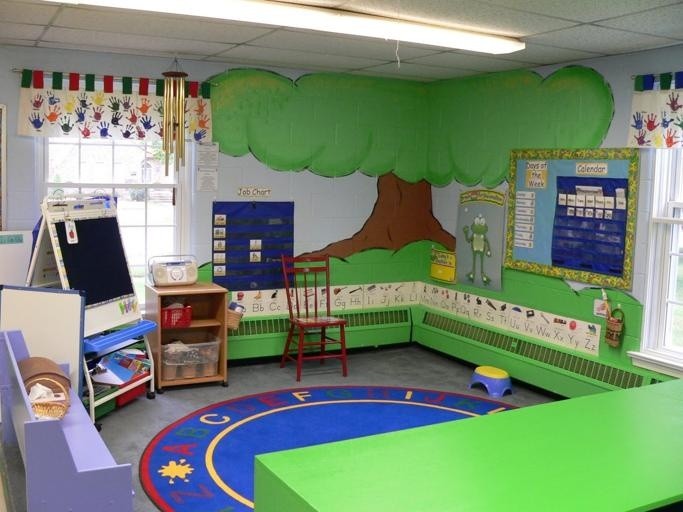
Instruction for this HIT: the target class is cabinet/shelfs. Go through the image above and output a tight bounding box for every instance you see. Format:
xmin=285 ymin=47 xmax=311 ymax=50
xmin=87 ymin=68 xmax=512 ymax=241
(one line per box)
xmin=144 ymin=284 xmax=229 ymax=393
xmin=82 ymin=330 xmax=156 ymax=424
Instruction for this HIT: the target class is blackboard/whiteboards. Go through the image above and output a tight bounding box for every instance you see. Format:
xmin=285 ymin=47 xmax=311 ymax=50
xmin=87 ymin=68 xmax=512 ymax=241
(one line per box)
xmin=49 ymin=215 xmax=134 ymax=310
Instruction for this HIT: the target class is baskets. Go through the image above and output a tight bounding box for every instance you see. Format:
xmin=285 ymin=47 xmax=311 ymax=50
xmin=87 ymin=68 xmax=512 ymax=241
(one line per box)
xmin=160 ymin=303 xmax=193 ymax=330
xmin=605 ymin=308 xmax=624 ymax=348
xmin=24 ymin=377 xmax=71 ymax=419
xmin=226 ymin=309 xmax=243 ymax=331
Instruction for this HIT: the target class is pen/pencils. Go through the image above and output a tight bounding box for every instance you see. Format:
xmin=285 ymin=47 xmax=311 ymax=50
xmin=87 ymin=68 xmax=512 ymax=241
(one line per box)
xmin=602 ymin=287 xmax=611 ymax=319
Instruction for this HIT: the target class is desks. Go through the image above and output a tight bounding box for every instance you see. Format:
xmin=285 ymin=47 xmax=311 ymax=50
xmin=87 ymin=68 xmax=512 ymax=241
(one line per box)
xmin=1 ymin=330 xmax=134 ymax=512
xmin=254 ymin=378 xmax=682 ymax=512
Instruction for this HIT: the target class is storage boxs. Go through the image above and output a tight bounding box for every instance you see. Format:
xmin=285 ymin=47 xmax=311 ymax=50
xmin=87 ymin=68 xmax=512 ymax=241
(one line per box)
xmin=114 ymin=371 xmax=149 ymax=406
xmin=162 ymin=330 xmax=221 ymax=380
xmin=82 ymin=385 xmax=118 ymax=419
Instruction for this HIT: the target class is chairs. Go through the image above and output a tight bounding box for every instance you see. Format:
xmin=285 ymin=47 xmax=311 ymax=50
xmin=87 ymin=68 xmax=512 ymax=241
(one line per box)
xmin=280 ymin=253 xmax=348 ymax=382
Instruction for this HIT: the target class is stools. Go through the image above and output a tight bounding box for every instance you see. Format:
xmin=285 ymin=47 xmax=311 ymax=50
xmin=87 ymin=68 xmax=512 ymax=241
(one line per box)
xmin=470 ymin=365 xmax=514 ymax=398
